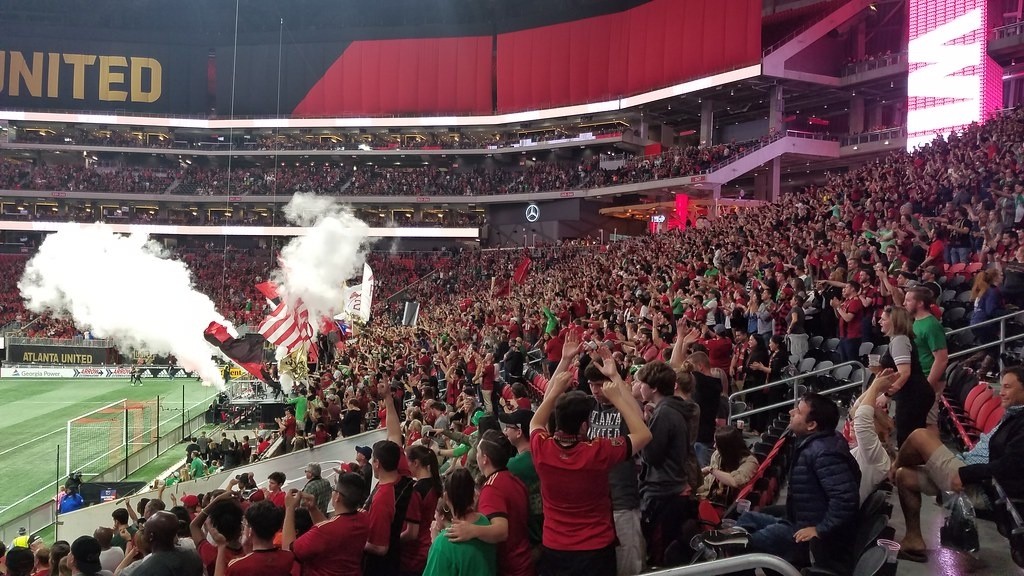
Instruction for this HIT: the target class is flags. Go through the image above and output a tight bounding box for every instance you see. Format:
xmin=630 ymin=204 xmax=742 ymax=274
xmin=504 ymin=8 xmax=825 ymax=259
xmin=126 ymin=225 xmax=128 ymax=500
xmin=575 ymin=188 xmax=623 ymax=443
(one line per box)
xmin=676 ymin=194 xmax=688 ymax=232
xmin=257 ymin=294 xmax=313 ymax=355
xmin=204 ymin=321 xmax=269 ymax=384
xmin=338 ymin=322 xmax=352 ymax=337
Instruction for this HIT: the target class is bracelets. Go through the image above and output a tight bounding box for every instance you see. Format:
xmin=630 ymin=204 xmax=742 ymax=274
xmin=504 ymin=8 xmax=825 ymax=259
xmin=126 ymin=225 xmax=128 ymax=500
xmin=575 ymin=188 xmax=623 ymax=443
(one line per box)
xmin=701 ymin=333 xmax=706 ymax=335
xmin=926 ymin=231 xmax=930 ymax=233
xmin=201 ymin=509 xmax=209 ymax=516
xmin=124 ymin=540 xmax=131 ymax=543
xmin=709 ymin=466 xmax=713 ymax=472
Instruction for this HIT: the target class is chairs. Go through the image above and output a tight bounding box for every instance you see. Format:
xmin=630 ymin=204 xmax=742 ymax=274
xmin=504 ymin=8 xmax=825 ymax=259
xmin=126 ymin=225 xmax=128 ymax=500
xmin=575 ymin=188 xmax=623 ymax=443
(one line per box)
xmin=511 ymin=261 xmax=1023 ymax=576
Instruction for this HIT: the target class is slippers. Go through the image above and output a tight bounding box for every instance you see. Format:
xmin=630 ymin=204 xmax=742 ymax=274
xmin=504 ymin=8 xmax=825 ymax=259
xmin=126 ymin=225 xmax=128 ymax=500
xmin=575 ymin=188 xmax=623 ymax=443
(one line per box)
xmin=898 ymin=550 xmax=928 ymax=562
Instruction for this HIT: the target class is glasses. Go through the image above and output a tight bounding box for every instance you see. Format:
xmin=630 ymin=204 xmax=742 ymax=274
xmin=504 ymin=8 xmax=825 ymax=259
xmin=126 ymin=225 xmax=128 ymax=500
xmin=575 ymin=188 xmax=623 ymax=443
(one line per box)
xmin=240 ymin=521 xmax=249 ymax=528
xmin=505 ymin=425 xmax=516 ymax=431
xmin=330 ymin=485 xmax=344 ymax=498
xmin=305 ymin=470 xmax=308 ymax=473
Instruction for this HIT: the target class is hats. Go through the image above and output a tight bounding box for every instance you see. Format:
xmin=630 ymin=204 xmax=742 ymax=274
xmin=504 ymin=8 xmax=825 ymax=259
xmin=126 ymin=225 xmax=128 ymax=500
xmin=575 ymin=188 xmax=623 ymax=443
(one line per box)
xmin=18 ymin=527 xmax=26 ymax=535
xmin=471 ymin=411 xmax=485 ymax=426
xmin=499 ymin=409 xmax=535 ymax=426
xmin=236 ymin=473 xmax=248 ymax=483
xmin=180 ymin=495 xmax=199 ymax=506
xmin=341 ymin=463 xmax=360 ymax=473
xmin=783 ymin=287 xmax=794 ymax=298
xmin=27 ymin=534 xmax=43 ymax=546
xmin=356 ymin=446 xmax=372 ymax=459
xmin=421 ymin=425 xmax=433 ymax=437
xmin=712 ymin=324 xmax=726 ymax=335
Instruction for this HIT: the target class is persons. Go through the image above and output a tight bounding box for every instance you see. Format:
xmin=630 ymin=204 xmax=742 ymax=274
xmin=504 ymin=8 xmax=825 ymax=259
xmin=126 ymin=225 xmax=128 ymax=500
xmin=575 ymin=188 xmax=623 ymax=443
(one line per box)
xmin=0 ymin=21 xmax=1024 ymax=576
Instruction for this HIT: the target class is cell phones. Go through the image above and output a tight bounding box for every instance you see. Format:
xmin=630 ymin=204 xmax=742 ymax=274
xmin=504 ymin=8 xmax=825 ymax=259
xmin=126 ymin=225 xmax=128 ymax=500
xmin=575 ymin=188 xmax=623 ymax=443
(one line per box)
xmin=427 ymin=431 xmax=438 ymax=438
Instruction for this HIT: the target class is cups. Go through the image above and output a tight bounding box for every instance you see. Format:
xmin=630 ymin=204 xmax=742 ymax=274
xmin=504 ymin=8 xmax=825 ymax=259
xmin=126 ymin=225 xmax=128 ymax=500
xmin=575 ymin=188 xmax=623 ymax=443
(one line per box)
xmin=868 ymin=354 xmax=880 ymax=373
xmin=689 ymin=535 xmax=718 ymax=562
xmin=734 ymin=499 xmax=752 ymax=514
xmin=737 ymin=420 xmax=744 ymax=429
xmin=721 ymin=519 xmax=736 ymax=529
xmin=876 ymin=539 xmax=901 ymax=563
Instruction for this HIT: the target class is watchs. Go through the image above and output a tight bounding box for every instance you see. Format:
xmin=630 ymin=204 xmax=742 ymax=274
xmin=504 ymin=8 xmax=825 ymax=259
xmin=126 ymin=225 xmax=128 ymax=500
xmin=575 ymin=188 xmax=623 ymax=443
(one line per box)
xmin=884 ymin=392 xmax=893 ymax=397
